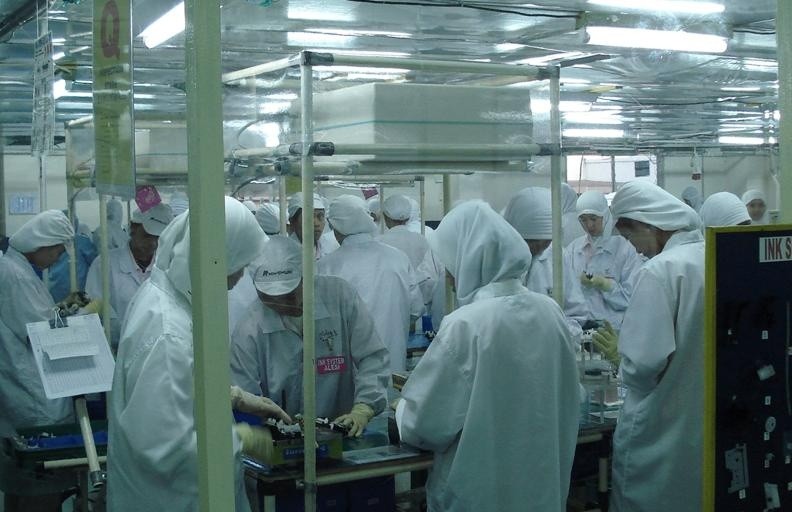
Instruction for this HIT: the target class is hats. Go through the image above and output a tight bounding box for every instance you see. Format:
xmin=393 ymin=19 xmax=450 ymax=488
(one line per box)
xmin=63 ymin=243 xmax=75 ymax=256
xmin=383 ymin=195 xmax=412 ymax=221
xmin=141 ymin=212 xmax=169 ymax=238
xmin=252 ymin=264 xmax=301 ymax=296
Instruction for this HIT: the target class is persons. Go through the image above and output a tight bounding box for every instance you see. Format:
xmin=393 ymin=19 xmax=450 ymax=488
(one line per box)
xmin=48 ymin=175 xmax=771 ymax=441
xmin=102 ymin=192 xmax=293 ymax=511
xmin=228 ymin=236 xmax=392 ymax=512
xmin=0 ymin=206 xmax=103 ymax=434
xmin=587 ymin=178 xmax=705 ymax=511
xmin=391 ymin=196 xmax=584 ymax=511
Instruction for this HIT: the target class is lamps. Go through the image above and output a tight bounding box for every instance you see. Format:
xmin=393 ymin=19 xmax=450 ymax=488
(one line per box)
xmin=575 ymin=12 xmax=736 ymax=57
xmin=132 ymin=2 xmax=186 ymax=51
xmin=49 ymin=78 xmax=73 ymax=100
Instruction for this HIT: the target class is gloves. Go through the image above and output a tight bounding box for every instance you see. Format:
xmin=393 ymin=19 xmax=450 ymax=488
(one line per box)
xmin=590 ymin=318 xmax=621 ymax=368
xmin=332 ymin=404 xmax=374 ymax=438
xmin=582 ymin=270 xmax=609 ymax=292
xmin=232 ymin=386 xmax=293 ymax=424
xmin=237 ymin=423 xmax=274 ymax=462
